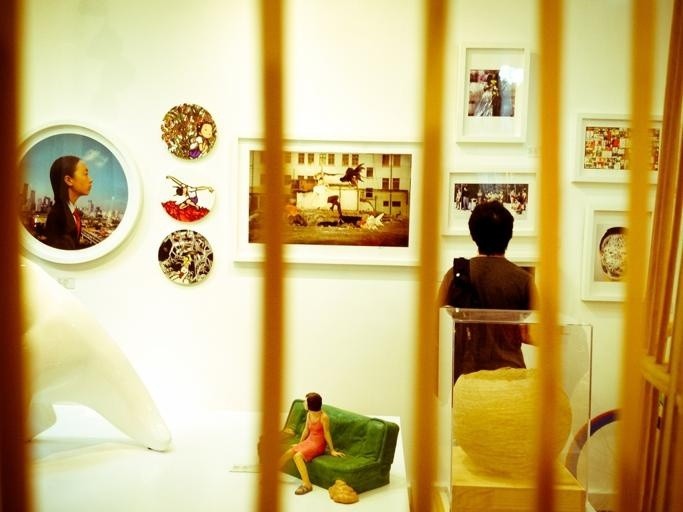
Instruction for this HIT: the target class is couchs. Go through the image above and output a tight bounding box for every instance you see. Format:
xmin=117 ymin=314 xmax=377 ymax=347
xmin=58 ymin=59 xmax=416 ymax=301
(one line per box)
xmin=257 ymin=398 xmax=400 ymax=494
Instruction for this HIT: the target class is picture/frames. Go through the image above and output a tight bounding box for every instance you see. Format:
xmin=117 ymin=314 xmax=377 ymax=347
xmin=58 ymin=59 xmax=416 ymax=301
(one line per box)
xmin=571 ymin=114 xmax=662 ymax=185
xmin=159 ymin=102 xmax=217 ymax=161
xmin=441 ymin=168 xmax=537 ymax=237
xmin=232 ymin=135 xmax=423 ymax=267
xmin=14 ymin=121 xmax=143 ymax=266
xmin=580 ymin=207 xmax=653 ymax=302
xmin=157 ymin=229 xmax=213 ymax=286
xmin=160 ymin=173 xmax=214 ymax=223
xmin=454 ymin=43 xmax=530 ymax=142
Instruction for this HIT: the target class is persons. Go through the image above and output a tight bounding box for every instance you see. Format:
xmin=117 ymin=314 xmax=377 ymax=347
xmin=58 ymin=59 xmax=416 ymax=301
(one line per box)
xmin=439 ymin=201 xmax=539 ymax=384
xmin=278 ymin=392 xmax=347 ymax=500
xmin=166 ymin=174 xmax=215 ymax=211
xmin=44 ymin=155 xmax=96 ymax=251
xmin=453 ymin=185 xmax=529 ymax=217
xmin=473 ymin=69 xmax=504 ymax=120
xmin=187 ymin=121 xmax=213 ymax=159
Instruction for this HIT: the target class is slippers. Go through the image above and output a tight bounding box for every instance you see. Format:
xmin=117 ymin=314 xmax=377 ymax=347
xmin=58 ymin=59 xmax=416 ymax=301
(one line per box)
xmin=293 ymin=485 xmax=313 ymax=495
